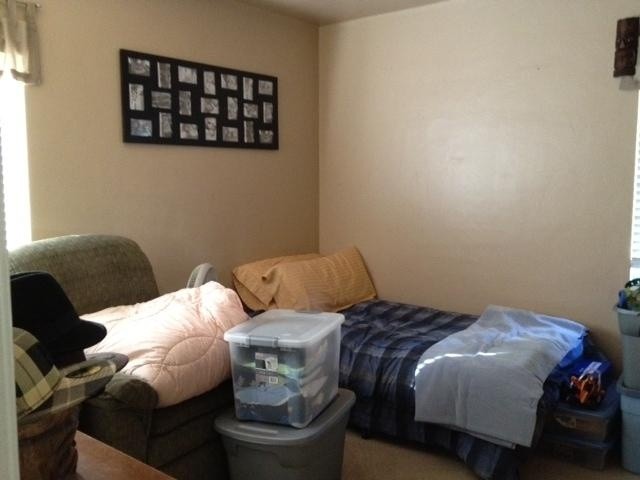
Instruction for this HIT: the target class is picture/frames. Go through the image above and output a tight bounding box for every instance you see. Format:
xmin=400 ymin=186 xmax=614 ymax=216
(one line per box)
xmin=118 ymin=48 xmax=277 ymax=150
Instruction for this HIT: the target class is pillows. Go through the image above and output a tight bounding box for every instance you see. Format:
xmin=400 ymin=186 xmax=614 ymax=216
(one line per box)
xmin=232 ymin=246 xmax=376 ymax=317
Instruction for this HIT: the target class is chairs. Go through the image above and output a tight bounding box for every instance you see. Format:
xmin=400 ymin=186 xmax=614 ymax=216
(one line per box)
xmin=5 ymin=235 xmax=231 ymax=467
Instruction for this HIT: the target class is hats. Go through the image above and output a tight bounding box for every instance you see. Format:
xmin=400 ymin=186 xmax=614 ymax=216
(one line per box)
xmin=10 ymin=273 xmax=128 ymax=420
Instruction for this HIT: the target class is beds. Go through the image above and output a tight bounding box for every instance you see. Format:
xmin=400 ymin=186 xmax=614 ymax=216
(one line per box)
xmin=230 ymin=244 xmax=613 ymax=480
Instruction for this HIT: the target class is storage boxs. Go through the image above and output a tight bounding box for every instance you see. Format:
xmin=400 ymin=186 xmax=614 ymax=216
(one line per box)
xmin=546 ymin=302 xmax=639 ymax=475
xmin=213 ymin=309 xmax=357 ymax=480
